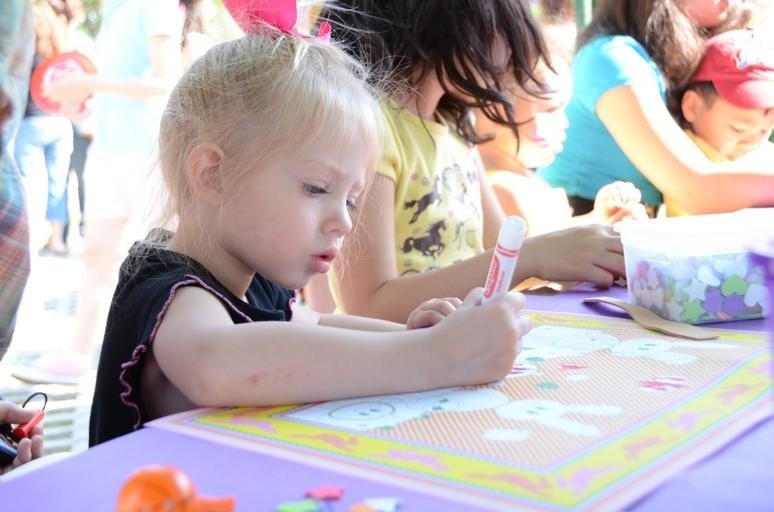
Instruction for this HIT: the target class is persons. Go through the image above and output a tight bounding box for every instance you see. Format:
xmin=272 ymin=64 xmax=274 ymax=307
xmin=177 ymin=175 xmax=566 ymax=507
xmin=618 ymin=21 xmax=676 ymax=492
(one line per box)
xmin=300 ymin=1 xmax=625 ymax=324
xmin=526 ymin=1 xmax=774 ymax=215
xmin=2 ymin=1 xmax=298 ymax=477
xmin=91 ymin=36 xmax=531 ymax=446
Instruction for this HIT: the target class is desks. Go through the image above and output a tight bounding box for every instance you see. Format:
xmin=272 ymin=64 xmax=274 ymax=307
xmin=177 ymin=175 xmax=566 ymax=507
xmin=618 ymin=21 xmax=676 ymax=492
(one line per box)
xmin=0 ymin=280 xmax=774 ymax=511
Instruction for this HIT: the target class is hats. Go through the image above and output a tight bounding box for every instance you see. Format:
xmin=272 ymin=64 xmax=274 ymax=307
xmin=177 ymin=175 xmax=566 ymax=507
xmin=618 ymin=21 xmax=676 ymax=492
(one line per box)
xmin=688 ymin=29 xmax=772 ymax=109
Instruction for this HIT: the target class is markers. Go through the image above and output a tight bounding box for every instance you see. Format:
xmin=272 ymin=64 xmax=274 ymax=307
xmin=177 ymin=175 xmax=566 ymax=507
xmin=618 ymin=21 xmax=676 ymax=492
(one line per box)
xmin=479 ymin=216 xmax=526 ymax=304
